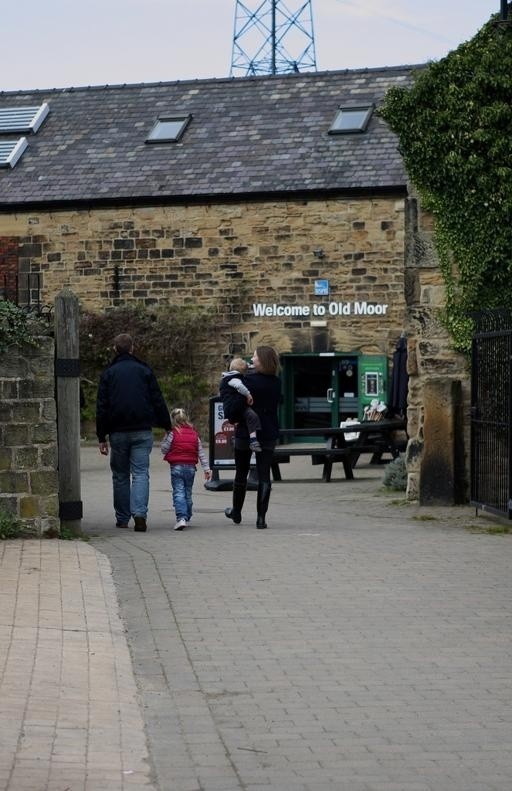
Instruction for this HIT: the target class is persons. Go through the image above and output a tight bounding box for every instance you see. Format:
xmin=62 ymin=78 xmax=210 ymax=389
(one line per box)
xmin=160 ymin=408 xmax=211 ymax=531
xmin=217 ymin=358 xmax=262 ymax=453
xmin=224 ymin=345 xmax=283 ymax=529
xmin=96 ymin=333 xmax=173 ymax=531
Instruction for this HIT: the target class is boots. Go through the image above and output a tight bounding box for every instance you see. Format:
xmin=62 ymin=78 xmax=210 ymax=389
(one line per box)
xmin=257 ymin=482 xmax=271 ymax=529
xmin=225 ymin=482 xmax=246 ymax=523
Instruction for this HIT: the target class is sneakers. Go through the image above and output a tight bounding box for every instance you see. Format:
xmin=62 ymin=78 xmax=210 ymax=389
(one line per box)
xmin=116 ymin=522 xmax=128 ymax=528
xmin=174 ymin=518 xmax=186 ymax=530
xmin=134 ymin=517 xmax=146 ymax=531
xmin=249 ymin=441 xmax=262 ymax=452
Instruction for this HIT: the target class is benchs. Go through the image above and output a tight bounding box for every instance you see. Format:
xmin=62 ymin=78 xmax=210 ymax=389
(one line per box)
xmin=269 ymin=415 xmax=410 ymax=484
